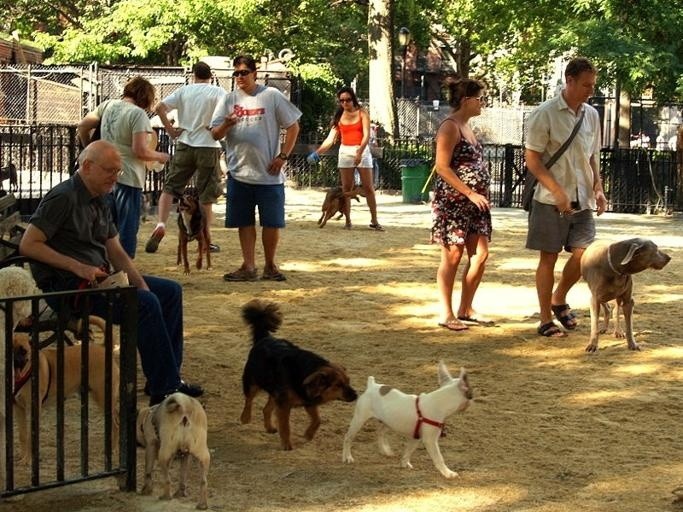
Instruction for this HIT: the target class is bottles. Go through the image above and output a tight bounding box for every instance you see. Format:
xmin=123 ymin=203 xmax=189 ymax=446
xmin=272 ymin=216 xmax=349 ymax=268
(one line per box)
xmin=306 ymin=150 xmax=320 ymax=165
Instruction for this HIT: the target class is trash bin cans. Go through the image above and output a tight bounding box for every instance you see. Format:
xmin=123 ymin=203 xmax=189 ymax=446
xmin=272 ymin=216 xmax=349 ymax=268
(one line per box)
xmin=400 ymin=159 xmax=432 ymax=204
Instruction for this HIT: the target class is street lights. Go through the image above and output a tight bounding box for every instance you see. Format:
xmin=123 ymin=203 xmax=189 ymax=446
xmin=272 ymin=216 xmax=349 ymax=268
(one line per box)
xmin=397 ymin=27 xmax=410 ymax=151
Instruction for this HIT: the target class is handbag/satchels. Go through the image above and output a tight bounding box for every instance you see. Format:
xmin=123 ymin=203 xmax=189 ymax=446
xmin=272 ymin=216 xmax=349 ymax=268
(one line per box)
xmin=521 ymin=172 xmax=538 ymax=211
xmin=88 ymin=100 xmax=110 ymax=144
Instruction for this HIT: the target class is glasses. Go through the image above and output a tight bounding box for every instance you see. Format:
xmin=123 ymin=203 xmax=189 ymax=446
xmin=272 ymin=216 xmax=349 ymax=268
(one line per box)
xmin=232 ymin=69 xmax=254 ymax=78
xmin=338 ymin=98 xmax=352 ymax=104
xmin=464 ymin=94 xmax=484 ymax=102
xmin=88 ymin=159 xmax=124 ymax=176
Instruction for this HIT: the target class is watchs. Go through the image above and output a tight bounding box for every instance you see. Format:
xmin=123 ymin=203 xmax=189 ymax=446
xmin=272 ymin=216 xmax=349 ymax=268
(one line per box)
xmin=278 ymin=153 xmax=288 ymax=161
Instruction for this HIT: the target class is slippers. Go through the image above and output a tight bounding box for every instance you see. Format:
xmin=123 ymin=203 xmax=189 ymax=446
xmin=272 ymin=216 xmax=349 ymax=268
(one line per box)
xmin=551 ymin=304 xmax=577 ymax=330
xmin=437 ymin=319 xmax=470 ymax=331
xmin=537 ymin=322 xmax=566 ymax=338
xmin=456 ymin=313 xmax=484 ymax=322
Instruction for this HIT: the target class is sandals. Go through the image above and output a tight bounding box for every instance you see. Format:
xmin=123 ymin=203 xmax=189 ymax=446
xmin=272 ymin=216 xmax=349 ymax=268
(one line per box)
xmin=345 ymin=221 xmax=353 ymax=230
xmin=368 ymin=221 xmax=384 ymax=231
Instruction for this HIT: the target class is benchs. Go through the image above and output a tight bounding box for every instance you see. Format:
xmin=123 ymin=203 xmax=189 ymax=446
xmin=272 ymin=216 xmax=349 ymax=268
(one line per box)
xmin=0 ymin=194 xmax=82 ymax=349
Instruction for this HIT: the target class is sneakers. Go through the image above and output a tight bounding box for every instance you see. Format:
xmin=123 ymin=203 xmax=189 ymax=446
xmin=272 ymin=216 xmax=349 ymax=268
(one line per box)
xmin=208 ymin=244 xmax=220 ymax=251
xmin=146 ymin=227 xmax=165 ymax=253
xmin=261 ymin=263 xmax=287 ymax=281
xmin=224 ymin=261 xmax=256 ymax=283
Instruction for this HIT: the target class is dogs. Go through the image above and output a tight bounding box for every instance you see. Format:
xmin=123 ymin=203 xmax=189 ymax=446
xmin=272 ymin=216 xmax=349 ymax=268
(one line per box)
xmin=177 ymin=194 xmax=213 ymax=276
xmin=13 ymin=315 xmax=120 ymax=468
xmin=342 ymin=360 xmax=474 ymax=480
xmin=113 ymin=392 xmax=211 ymax=510
xmin=0 ymin=264 xmax=43 ymax=502
xmin=240 ymin=300 xmax=358 ymax=451
xmin=0 ymin=167 xmax=18 ymax=192
xmin=580 ymin=238 xmax=671 ymax=353
xmin=318 ymin=186 xmax=360 ymax=230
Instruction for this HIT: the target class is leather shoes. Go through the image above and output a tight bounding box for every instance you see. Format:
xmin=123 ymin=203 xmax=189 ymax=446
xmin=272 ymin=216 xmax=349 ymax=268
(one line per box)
xmin=143 ymin=379 xmax=203 ymax=407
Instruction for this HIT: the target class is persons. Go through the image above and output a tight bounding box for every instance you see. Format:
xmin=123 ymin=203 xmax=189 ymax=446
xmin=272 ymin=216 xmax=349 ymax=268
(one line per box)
xmin=317 ymin=86 xmax=387 ymax=231
xmin=18 ymin=139 xmax=204 ymax=407
xmin=77 ymin=76 xmax=172 ymax=260
xmin=430 ymin=77 xmax=493 ymax=330
xmin=208 ymin=55 xmax=304 ymax=281
xmin=524 ymin=59 xmax=608 ymax=336
xmin=144 ymin=62 xmax=230 ymax=255
